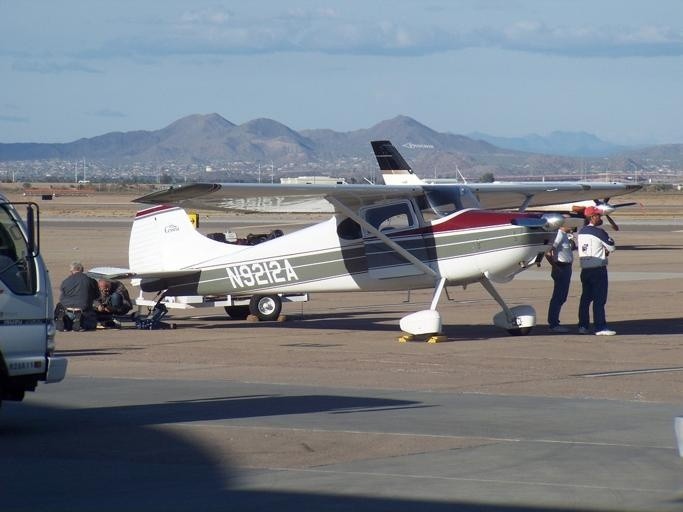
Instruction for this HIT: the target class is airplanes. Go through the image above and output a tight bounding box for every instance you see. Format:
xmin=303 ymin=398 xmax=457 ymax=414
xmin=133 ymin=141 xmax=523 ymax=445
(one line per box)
xmin=85 ymin=140 xmax=642 ymax=343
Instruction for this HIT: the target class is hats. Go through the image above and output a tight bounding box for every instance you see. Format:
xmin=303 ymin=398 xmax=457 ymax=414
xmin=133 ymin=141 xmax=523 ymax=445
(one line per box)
xmin=584 ymin=206 xmax=603 ymax=216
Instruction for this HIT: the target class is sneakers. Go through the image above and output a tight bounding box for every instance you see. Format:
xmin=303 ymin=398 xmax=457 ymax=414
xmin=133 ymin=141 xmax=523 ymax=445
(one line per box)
xmin=578 ymin=327 xmax=589 ymax=335
xmin=549 ymin=326 xmax=570 ymax=333
xmin=73 ymin=313 xmax=81 ymax=331
xmin=57 ymin=312 xmax=65 ymax=331
xmin=592 ymin=329 xmax=616 ymax=335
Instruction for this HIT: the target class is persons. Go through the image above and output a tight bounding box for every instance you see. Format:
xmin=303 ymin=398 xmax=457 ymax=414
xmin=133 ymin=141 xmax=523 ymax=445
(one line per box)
xmin=53 ymin=261 xmax=100 ymax=333
xmin=544 ymin=218 xmax=573 ymax=333
xmin=95 ymin=278 xmax=133 ymax=329
xmin=575 ymin=204 xmax=618 ymax=335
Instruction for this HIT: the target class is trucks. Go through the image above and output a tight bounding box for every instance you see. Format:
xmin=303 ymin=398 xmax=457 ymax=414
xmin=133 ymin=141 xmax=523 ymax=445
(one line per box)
xmin=0 ymin=195 xmax=69 ymax=404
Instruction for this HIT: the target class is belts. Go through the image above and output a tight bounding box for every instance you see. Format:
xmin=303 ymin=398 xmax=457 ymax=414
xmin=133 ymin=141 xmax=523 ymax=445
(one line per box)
xmin=67 ymin=310 xmax=81 ymax=313
